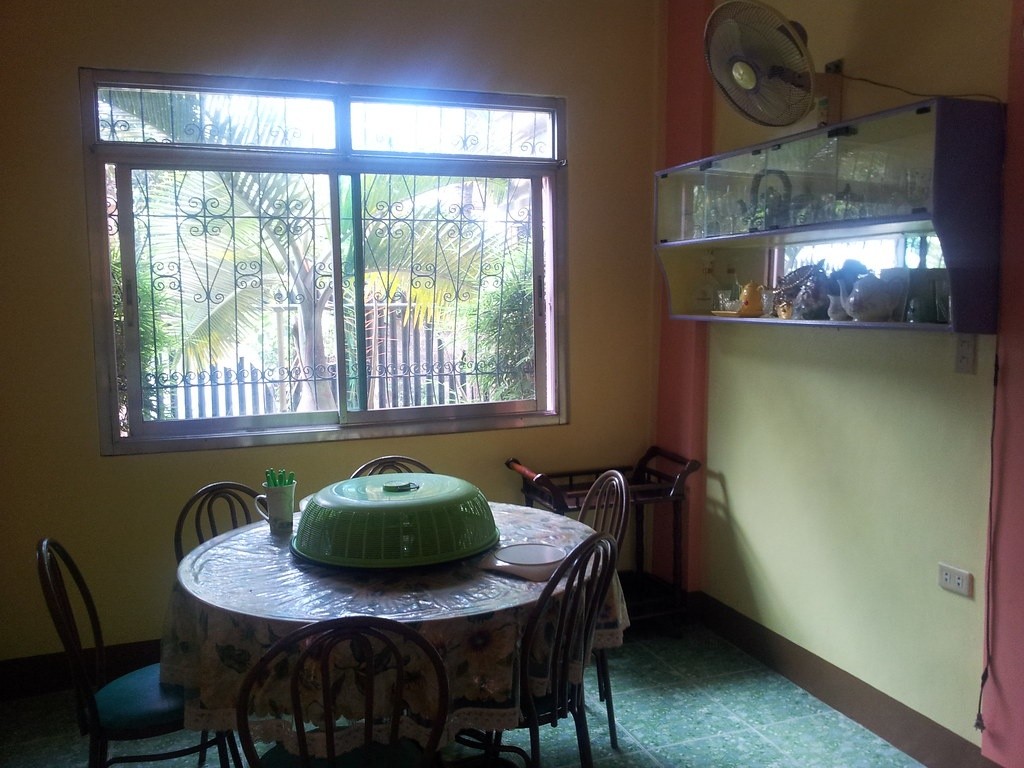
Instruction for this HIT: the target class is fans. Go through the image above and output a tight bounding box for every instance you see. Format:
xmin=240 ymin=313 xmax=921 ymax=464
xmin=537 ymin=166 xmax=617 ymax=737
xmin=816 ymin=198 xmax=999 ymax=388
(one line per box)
xmin=703 ymin=0 xmax=845 ymax=130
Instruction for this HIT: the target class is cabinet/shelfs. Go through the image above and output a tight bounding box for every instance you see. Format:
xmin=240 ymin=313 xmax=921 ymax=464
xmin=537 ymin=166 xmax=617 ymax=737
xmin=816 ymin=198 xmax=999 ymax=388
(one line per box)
xmin=651 ymin=94 xmax=1006 ymax=335
xmin=505 ymin=446 xmax=703 ymax=639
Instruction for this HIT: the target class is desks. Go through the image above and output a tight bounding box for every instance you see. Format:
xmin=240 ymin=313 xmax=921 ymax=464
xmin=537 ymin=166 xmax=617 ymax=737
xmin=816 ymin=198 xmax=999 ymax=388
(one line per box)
xmin=177 ymin=501 xmax=612 ymax=768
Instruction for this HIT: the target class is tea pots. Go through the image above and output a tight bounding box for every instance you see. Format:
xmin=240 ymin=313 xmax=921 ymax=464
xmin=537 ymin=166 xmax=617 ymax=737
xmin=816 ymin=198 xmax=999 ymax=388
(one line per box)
xmin=738 ymin=169 xmax=791 ymax=230
xmin=837 ymin=269 xmax=902 ymax=323
xmin=739 ymin=280 xmax=766 ymax=316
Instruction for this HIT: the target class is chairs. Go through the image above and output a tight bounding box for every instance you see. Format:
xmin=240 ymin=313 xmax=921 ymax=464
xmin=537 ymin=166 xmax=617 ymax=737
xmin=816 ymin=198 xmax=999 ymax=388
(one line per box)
xmin=407 ymin=531 xmax=617 ymax=768
xmin=492 ymin=468 xmax=630 ymax=758
xmin=35 ymin=536 xmax=245 ymax=768
xmin=352 ymin=455 xmax=434 ymax=478
xmin=173 ymin=481 xmax=270 ymax=768
xmin=236 ymin=615 xmax=449 ymax=768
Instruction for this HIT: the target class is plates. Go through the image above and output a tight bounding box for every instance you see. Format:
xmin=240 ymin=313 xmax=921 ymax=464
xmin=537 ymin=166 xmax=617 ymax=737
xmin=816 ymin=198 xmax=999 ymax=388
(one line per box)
xmin=479 ymin=544 xmax=567 ymax=581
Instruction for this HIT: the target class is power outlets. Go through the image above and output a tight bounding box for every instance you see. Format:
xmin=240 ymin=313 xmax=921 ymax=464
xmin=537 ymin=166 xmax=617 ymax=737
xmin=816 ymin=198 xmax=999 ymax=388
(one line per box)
xmin=954 ymin=332 xmax=978 ymax=374
xmin=937 ymin=562 xmax=974 ymax=597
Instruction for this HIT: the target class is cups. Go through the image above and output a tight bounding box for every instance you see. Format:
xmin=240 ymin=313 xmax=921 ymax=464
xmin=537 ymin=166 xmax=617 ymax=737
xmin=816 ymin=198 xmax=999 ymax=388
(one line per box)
xmin=717 ymin=289 xmax=732 ymax=311
xmin=761 ymin=291 xmax=775 ymax=317
xmin=827 ymin=294 xmax=846 ymax=321
xmin=255 ymin=480 xmax=296 ymax=532
xmin=724 ymin=300 xmax=741 ymax=312
